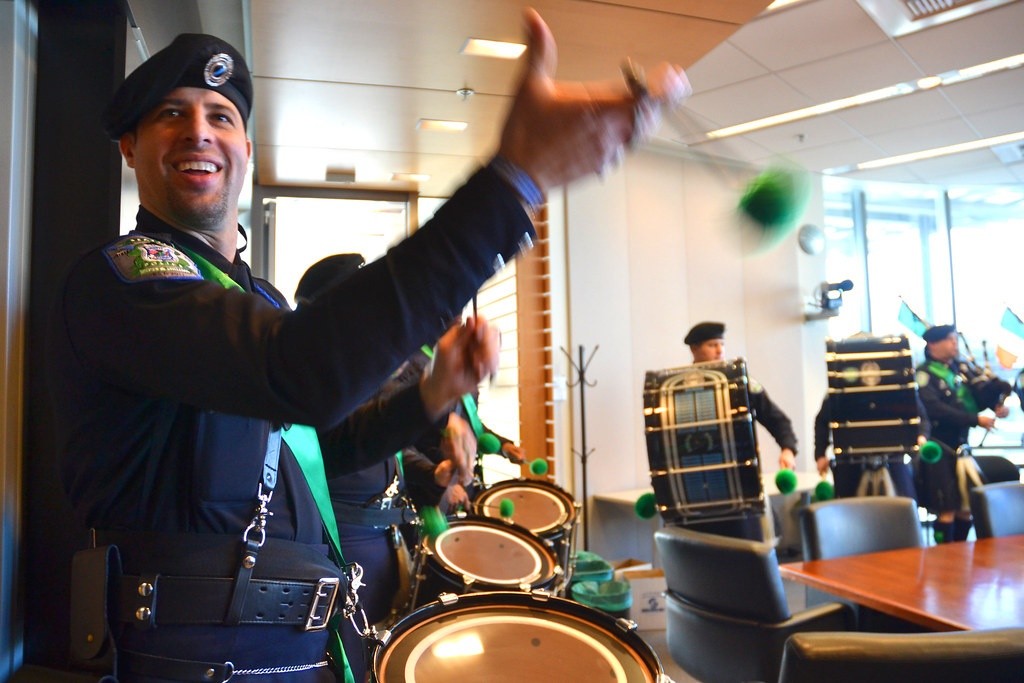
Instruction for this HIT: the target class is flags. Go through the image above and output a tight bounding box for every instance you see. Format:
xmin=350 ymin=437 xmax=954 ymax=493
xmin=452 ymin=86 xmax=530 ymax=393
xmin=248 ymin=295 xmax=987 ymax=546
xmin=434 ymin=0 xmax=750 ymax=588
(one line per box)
xmin=995 ymin=309 xmax=1024 ymax=370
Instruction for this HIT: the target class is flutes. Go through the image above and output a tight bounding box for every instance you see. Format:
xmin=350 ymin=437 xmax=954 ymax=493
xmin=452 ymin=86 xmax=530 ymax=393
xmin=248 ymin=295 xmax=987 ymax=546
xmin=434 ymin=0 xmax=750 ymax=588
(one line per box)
xmin=953 ymin=331 xmax=1023 ymax=413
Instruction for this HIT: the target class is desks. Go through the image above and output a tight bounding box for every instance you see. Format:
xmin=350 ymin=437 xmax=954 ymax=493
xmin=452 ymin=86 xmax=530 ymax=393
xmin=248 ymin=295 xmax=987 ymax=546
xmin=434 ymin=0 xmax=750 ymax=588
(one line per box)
xmin=777 ymin=530 xmax=1024 ymax=632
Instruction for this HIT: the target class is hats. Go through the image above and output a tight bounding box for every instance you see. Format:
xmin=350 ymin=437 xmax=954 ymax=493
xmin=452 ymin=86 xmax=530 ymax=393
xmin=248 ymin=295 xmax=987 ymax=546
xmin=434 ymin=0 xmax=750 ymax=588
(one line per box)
xmin=98 ymin=33 xmax=252 ymax=141
xmin=294 ymin=253 xmax=366 ymax=307
xmin=923 ymin=324 xmax=956 ymax=343
xmin=684 ymin=322 xmax=725 ymax=345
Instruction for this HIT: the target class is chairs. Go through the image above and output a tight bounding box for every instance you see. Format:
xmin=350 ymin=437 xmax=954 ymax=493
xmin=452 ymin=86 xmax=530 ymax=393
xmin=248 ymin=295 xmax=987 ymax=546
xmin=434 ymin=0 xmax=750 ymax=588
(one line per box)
xmin=652 ymin=452 xmax=1024 ymax=683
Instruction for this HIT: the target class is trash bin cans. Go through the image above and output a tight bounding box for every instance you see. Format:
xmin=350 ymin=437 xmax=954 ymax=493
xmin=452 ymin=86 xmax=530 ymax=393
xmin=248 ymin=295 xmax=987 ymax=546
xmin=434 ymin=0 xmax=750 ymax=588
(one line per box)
xmin=566 ymin=550 xmax=615 ymax=599
xmin=571 ymin=579 xmax=631 ymax=620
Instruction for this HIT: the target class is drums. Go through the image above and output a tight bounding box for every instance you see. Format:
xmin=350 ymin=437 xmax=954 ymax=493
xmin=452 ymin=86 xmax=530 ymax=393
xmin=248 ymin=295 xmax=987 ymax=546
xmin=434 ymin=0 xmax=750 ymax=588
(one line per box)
xmin=641 ymin=356 xmax=771 ymax=530
xmin=411 ymin=512 xmax=561 ymax=614
xmin=825 ymin=329 xmax=922 ymax=467
xmin=470 ymin=476 xmax=575 ymax=592
xmin=369 ymin=589 xmax=664 ymax=683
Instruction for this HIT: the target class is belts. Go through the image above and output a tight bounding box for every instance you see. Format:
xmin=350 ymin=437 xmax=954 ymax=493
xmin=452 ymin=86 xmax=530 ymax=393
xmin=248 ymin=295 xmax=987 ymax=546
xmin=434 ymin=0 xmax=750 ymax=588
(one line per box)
xmin=103 ymin=572 xmax=346 ymax=631
xmin=338 ymin=502 xmax=418 ymax=528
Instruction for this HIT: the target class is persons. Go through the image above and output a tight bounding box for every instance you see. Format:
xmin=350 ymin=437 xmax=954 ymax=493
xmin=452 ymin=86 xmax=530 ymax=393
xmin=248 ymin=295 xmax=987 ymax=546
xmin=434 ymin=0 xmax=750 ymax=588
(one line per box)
xmin=914 ymin=325 xmax=1009 ymax=545
xmin=684 ymin=322 xmax=799 ymax=473
xmin=23 ymin=3 xmax=652 ymax=683
xmin=296 ymin=251 xmax=526 ymax=683
xmin=814 ymin=332 xmax=917 ymax=504
